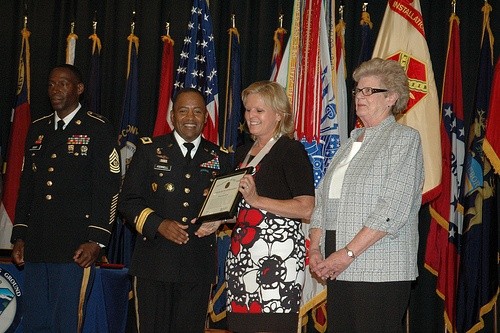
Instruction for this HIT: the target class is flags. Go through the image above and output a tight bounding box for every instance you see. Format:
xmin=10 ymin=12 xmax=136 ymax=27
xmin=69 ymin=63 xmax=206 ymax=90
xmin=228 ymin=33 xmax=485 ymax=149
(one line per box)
xmin=0 ymin=0 xmax=500 ymax=333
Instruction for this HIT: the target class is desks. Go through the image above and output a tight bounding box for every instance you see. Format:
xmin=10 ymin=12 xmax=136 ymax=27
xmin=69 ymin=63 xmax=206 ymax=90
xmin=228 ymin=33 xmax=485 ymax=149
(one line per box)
xmin=0 ymin=258 xmax=132 ymax=333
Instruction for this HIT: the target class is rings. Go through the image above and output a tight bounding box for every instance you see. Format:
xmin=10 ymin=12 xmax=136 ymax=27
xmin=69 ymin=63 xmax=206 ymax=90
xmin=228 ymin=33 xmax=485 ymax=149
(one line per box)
xmin=247 ymin=185 xmax=248 ymax=188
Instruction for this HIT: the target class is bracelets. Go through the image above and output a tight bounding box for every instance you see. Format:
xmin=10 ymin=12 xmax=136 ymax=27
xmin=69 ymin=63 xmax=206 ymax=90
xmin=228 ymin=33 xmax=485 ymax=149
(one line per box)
xmin=225 ymin=218 xmax=227 ymax=223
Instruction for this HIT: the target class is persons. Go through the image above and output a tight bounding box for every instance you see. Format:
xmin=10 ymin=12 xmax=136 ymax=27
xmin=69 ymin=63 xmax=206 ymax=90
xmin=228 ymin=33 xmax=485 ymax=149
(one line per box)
xmin=10 ymin=64 xmax=121 ymax=333
xmin=116 ymin=88 xmax=231 ymax=333
xmin=191 ymin=80 xmax=315 ymax=332
xmin=308 ymin=58 xmax=424 ymax=333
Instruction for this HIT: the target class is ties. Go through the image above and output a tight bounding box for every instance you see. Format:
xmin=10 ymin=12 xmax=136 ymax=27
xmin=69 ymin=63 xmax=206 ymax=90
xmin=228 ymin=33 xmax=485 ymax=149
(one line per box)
xmin=57 ymin=120 xmax=65 ymax=131
xmin=183 ymin=143 xmax=195 ymax=159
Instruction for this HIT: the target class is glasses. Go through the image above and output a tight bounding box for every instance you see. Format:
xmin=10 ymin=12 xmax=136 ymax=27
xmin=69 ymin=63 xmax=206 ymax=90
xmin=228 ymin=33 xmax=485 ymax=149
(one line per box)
xmin=351 ymin=86 xmax=388 ymax=96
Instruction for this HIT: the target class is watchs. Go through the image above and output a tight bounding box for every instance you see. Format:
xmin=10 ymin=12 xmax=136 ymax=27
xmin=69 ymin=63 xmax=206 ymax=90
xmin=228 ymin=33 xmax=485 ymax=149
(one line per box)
xmin=345 ymin=246 xmax=355 ymax=258
